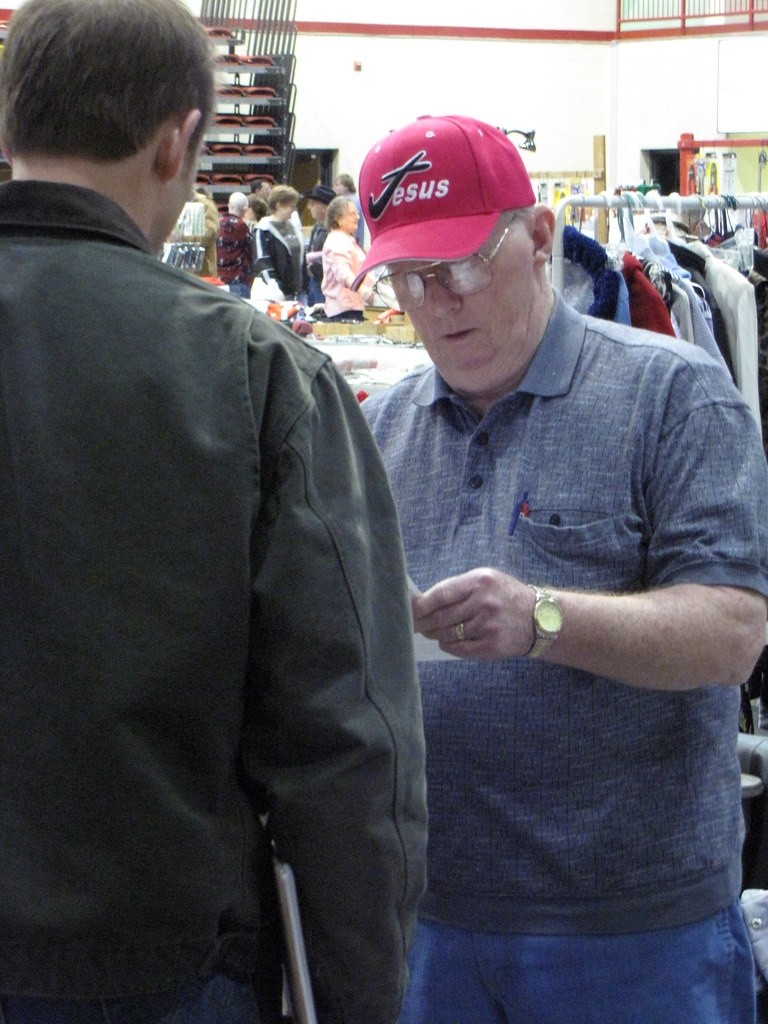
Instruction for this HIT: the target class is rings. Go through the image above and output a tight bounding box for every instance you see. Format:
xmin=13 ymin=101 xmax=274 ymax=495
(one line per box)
xmin=454 ymin=623 xmax=466 ymax=641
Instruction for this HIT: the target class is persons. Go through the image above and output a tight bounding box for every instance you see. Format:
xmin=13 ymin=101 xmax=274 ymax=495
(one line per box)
xmin=216 ymin=192 xmax=253 ymax=298
xmin=244 ymin=177 xmax=301 ymax=235
xmin=322 ymin=196 xmax=377 ymax=324
xmin=166 ymin=190 xmax=222 ymax=277
xmin=253 ymin=184 xmax=304 ymax=324
xmin=302 ymin=186 xmax=336 ymax=306
xmin=0 ymin=0 xmax=429 ymax=1024
xmin=356 ymin=114 xmax=768 ymax=1024
xmin=333 ymin=174 xmax=365 ymax=250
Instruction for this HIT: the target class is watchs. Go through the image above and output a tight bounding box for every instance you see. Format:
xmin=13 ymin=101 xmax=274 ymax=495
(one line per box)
xmin=522 ymin=583 xmax=566 ymax=660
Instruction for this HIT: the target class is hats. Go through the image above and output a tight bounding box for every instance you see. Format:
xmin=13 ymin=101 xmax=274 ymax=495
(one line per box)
xmin=347 ymin=115 xmax=540 ymax=293
xmin=307 ymin=185 xmax=336 ymax=205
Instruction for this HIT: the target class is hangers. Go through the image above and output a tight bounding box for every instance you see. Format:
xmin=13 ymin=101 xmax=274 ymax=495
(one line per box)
xmin=563 ymin=189 xmax=768 ymax=302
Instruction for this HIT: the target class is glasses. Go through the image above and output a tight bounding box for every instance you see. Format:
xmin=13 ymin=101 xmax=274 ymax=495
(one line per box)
xmin=372 ymin=213 xmax=522 ymax=315
